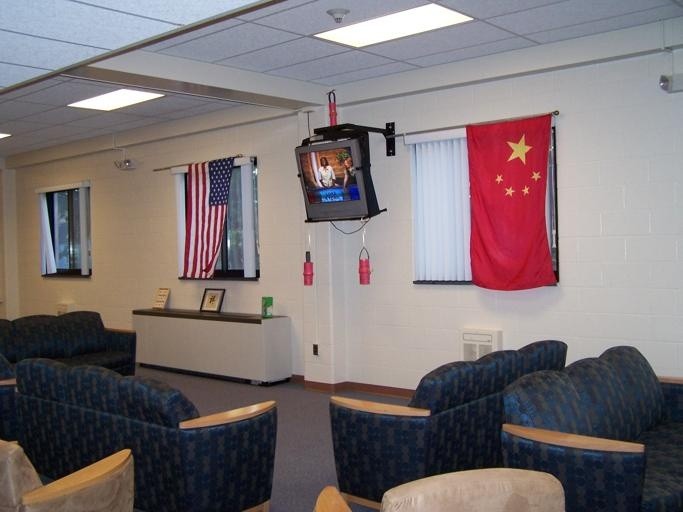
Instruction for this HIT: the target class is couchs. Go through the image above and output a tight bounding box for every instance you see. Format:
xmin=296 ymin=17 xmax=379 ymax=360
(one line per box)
xmin=2 ymin=312 xmax=137 ymax=389
xmin=328 ymin=340 xmax=567 ymax=508
xmin=15 ymin=356 xmax=277 ymax=512
xmin=501 ymin=346 xmax=682 ymax=512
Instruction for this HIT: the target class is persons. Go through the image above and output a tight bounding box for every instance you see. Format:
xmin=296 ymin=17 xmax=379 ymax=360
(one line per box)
xmin=318 ymin=157 xmax=357 ymax=189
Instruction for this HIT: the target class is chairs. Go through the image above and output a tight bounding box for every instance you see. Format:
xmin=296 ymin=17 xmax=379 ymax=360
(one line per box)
xmin=0 ymin=440 xmax=134 ymax=512
xmin=316 ymin=468 xmax=565 ymax=511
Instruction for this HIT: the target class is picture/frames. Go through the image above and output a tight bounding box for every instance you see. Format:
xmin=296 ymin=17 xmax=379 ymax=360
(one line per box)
xmin=200 ymin=288 xmax=226 ymax=313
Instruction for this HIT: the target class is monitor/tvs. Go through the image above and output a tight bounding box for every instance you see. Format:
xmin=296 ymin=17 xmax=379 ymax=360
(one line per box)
xmin=295 ymin=137 xmax=379 ymax=221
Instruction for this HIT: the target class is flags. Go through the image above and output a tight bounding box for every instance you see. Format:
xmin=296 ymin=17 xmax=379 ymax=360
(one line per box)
xmin=179 ymin=157 xmax=234 ymax=280
xmin=466 ymin=116 xmax=557 ymax=292
